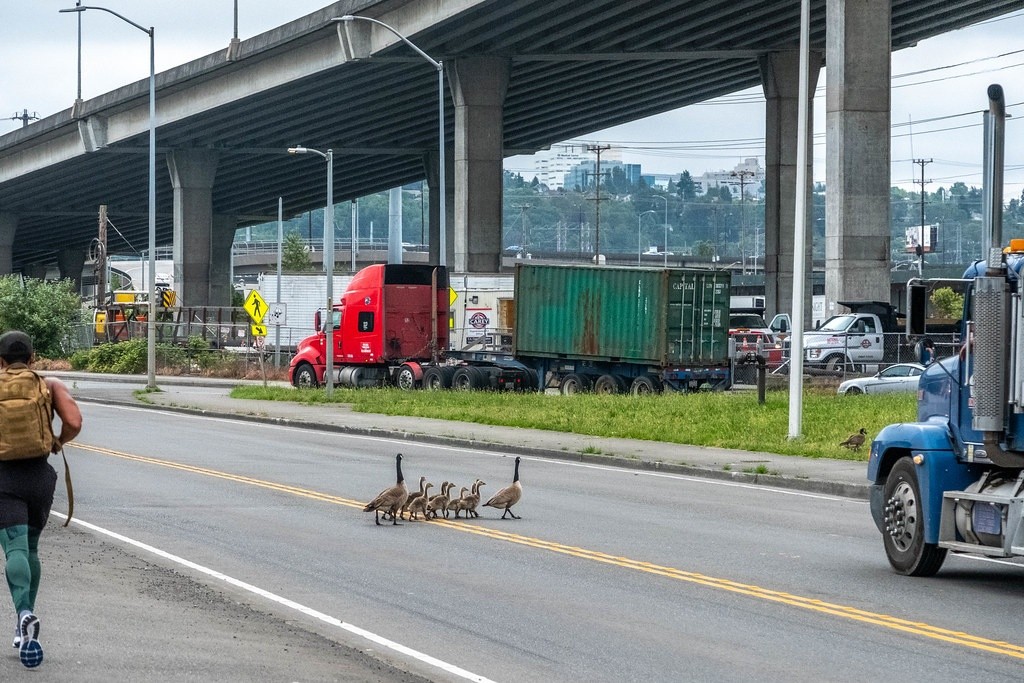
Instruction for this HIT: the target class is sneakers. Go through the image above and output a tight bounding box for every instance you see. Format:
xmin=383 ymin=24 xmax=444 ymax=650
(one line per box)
xmin=14 ymin=610 xmax=43 ymax=669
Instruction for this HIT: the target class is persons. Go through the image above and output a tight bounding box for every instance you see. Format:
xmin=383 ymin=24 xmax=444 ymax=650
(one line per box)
xmin=959 ymin=323 xmax=976 ymax=358
xmin=0 ymin=330 xmax=83 ymax=668
xmin=924 ymin=342 xmax=935 ymax=366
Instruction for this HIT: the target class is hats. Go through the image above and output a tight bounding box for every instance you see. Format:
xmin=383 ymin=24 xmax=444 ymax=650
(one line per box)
xmin=0 ymin=330 xmax=34 ymax=355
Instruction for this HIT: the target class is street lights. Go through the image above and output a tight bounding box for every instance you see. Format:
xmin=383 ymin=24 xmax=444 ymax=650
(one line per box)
xmin=331 ymin=14 xmax=448 ymax=264
xmin=58 ymin=4 xmax=160 ymax=393
xmin=286 ymin=144 xmax=337 ymax=389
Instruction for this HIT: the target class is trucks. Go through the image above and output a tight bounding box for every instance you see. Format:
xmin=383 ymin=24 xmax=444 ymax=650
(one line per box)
xmin=866 ymin=85 xmax=1024 ymax=577
xmin=290 ymin=263 xmax=538 ymax=395
xmin=780 ymin=300 xmax=912 ymax=377
xmin=513 ymin=263 xmax=729 ymax=395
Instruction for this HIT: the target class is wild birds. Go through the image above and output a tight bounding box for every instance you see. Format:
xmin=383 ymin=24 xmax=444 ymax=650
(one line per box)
xmin=839 ymin=427 xmax=867 ymax=451
xmin=362 ymin=453 xmax=486 ymax=525
xmin=482 ymin=457 xmax=522 ymax=520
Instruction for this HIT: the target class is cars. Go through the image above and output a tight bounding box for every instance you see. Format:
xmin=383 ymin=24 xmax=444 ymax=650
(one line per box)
xmin=729 ymin=313 xmax=779 ymax=361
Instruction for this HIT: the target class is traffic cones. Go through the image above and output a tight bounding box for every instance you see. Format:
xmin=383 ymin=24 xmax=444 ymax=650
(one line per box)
xmin=756 ymin=336 xmax=762 ymax=352
xmin=742 ymin=337 xmax=748 ymax=353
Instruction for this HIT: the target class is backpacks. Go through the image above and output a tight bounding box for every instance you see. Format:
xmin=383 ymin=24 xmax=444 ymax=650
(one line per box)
xmin=0 ymin=363 xmax=54 ymax=460
